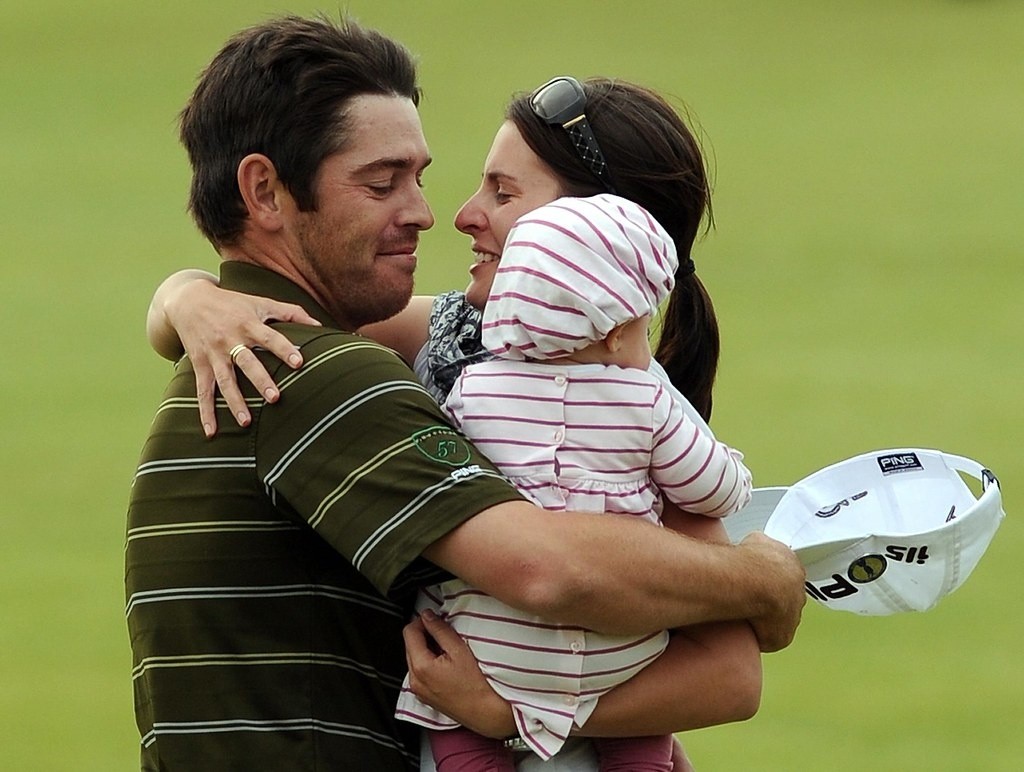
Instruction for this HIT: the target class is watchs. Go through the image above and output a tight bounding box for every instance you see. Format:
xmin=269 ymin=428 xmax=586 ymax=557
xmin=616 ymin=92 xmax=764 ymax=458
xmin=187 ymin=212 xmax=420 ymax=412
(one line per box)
xmin=499 ymin=737 xmax=533 ymax=752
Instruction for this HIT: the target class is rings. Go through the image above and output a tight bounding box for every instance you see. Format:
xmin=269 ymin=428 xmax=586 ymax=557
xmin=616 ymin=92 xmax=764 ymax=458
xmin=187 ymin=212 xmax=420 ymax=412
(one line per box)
xmin=230 ymin=344 xmax=250 ymax=361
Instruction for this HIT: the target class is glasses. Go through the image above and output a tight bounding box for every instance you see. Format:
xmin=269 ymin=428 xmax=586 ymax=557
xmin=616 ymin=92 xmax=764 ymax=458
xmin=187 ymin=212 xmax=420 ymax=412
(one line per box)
xmin=528 ymin=77 xmax=618 ymax=193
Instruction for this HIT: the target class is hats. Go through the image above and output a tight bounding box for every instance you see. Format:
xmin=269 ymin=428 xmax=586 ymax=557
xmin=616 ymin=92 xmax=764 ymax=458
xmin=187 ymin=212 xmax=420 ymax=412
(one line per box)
xmin=722 ymin=447 xmax=1007 ymax=618
xmin=481 ymin=192 xmax=680 ymax=361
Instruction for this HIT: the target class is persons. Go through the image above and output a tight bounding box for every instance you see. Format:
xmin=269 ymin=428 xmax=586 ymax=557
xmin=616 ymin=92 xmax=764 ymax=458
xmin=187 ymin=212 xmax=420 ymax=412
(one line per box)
xmin=393 ymin=194 xmax=752 ymax=771
xmin=124 ymin=7 xmax=806 ymax=772
xmin=146 ymin=76 xmax=761 ymax=771
